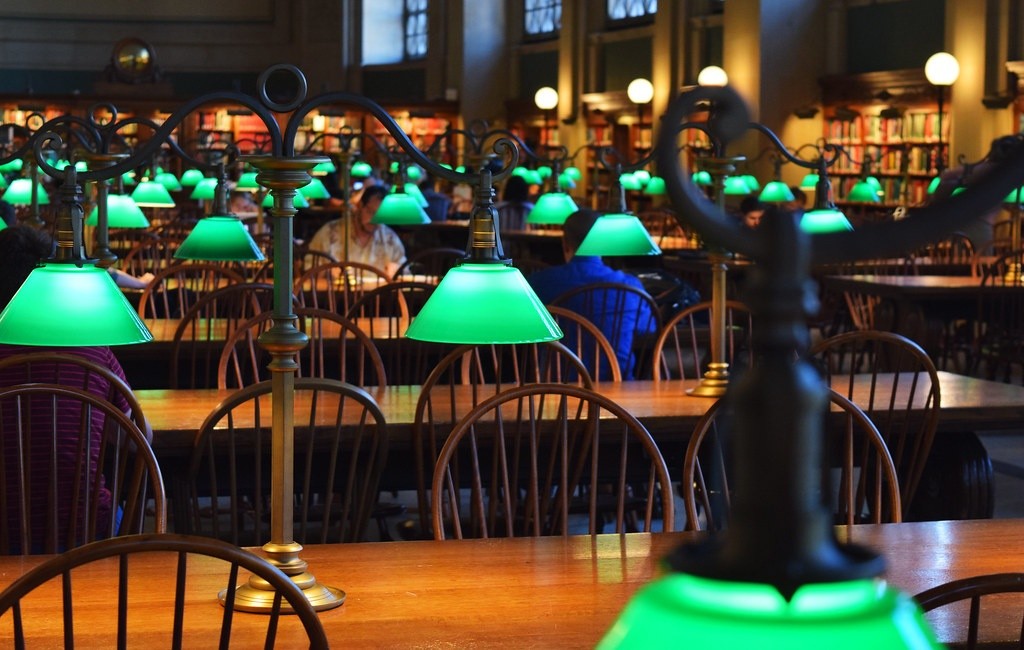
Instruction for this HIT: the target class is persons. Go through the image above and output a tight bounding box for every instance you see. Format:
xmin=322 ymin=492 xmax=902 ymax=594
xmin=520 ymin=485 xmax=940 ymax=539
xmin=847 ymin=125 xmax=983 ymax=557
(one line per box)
xmin=722 ymin=185 xmax=809 ymax=264
xmin=173 ymin=142 xmax=546 ymax=277
xmin=514 ymin=210 xmax=659 ymax=383
xmin=0 ymin=226 xmax=155 ymax=551
xmin=940 ymin=136 xmax=1014 ymax=256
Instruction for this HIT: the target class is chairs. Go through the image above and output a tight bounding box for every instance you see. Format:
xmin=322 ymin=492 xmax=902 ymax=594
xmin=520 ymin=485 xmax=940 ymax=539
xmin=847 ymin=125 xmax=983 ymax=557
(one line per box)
xmin=1 ymin=208 xmax=1024 ymax=650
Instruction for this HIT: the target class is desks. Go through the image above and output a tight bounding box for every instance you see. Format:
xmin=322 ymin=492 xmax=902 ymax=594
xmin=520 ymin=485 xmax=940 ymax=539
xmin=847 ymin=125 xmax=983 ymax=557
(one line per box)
xmin=823 ymin=273 xmax=1024 ymax=371
xmin=123 ymin=315 xmax=419 ymax=390
xmin=119 ymin=275 xmax=439 ymax=294
xmin=129 ymin=371 xmax=1024 ymax=497
xmin=0 ymin=518 xmax=1024 ymax=650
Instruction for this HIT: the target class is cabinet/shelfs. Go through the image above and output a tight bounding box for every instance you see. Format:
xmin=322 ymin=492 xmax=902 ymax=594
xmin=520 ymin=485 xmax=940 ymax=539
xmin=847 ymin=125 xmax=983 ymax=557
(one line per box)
xmin=502 ymin=94 xmax=558 ymax=174
xmin=362 ymin=109 xmax=458 ymax=167
xmin=193 ymin=108 xmax=273 ymax=156
xmin=294 ymin=110 xmax=360 ymax=156
xmin=580 ymin=79 xmax=709 ymax=210
xmin=822 ymin=67 xmax=950 ymax=209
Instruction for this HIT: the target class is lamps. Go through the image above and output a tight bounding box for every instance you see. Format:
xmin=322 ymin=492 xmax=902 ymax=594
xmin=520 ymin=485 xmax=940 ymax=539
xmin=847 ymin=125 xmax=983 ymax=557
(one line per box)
xmin=0 ymin=66 xmax=564 ymax=613
xmin=574 ymin=63 xmax=1024 ymax=650
